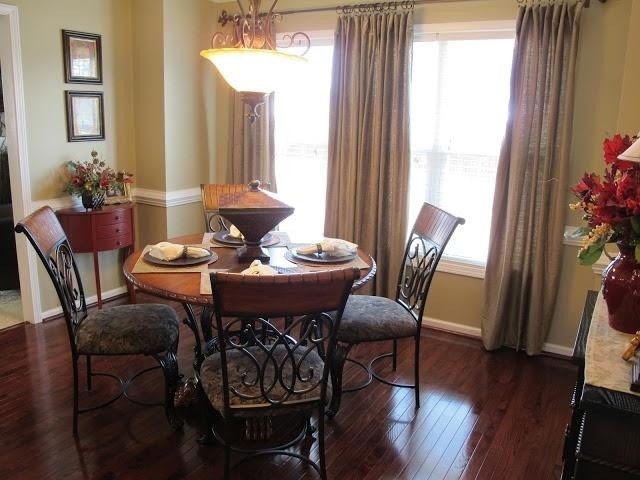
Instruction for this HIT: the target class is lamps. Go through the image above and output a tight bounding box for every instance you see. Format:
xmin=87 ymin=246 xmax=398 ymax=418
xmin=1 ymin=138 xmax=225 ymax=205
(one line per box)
xmin=199 ymin=6 xmax=311 ymax=94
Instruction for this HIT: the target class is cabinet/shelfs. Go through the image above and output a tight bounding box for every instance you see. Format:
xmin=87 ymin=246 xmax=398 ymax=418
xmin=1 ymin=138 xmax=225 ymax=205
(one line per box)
xmin=55 ymin=203 xmax=135 ymax=310
xmin=560 ymin=282 xmax=640 ymax=480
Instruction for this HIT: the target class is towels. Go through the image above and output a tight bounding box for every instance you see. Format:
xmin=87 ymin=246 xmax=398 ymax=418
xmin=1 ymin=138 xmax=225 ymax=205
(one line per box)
xmin=148 ymin=241 xmax=211 ymax=261
xmin=296 ymin=237 xmax=359 ymax=257
xmin=227 ymin=224 xmax=245 ymax=240
xmin=241 ymin=260 xmax=279 ymax=275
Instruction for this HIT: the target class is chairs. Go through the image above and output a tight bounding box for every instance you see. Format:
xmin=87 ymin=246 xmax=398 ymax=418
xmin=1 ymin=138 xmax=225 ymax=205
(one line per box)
xmin=13 ymin=204 xmax=185 ymax=432
xmin=324 ymin=203 xmax=465 ymax=419
xmin=200 ymin=183 xmax=294 ymax=343
xmin=198 ymin=268 xmax=355 ymax=479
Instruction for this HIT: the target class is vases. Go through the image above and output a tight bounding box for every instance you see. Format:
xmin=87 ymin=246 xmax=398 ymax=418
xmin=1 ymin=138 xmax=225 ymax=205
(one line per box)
xmin=82 ymin=189 xmax=104 ymax=211
xmin=602 ymin=241 xmax=640 ymax=333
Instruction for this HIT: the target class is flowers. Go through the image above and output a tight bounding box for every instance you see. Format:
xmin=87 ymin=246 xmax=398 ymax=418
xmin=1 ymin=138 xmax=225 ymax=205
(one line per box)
xmin=63 ymin=151 xmax=136 ymax=197
xmin=574 ymin=130 xmax=639 ymax=265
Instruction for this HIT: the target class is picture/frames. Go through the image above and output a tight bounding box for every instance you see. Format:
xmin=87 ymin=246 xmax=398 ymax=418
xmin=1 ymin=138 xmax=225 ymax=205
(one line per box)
xmin=61 ymin=29 xmax=104 ymax=85
xmin=65 ymin=90 xmax=105 ymax=142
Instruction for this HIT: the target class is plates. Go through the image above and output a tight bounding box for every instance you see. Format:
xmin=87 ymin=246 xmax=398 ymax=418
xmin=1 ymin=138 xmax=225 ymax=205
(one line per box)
xmin=290 ymin=246 xmax=358 ymax=263
xmin=214 ymin=230 xmax=273 ymax=244
xmin=143 ymin=246 xmax=214 ymax=266
